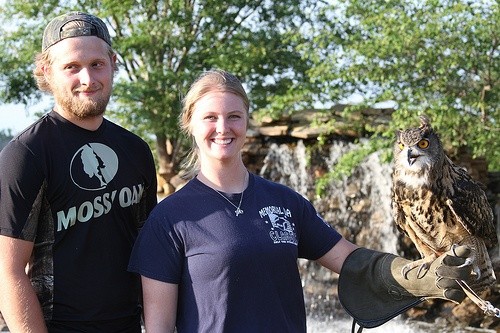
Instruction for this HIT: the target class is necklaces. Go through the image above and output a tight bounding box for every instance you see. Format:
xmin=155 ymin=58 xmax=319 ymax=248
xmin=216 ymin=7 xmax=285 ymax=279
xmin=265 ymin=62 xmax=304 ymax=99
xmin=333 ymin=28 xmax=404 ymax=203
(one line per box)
xmin=199 ymin=170 xmax=248 ymax=216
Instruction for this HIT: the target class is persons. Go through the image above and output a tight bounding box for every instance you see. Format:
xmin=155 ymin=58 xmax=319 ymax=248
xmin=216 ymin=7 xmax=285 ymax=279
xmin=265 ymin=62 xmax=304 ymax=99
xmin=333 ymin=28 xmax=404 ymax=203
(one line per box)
xmin=0 ymin=11 xmax=158 ymax=333
xmin=126 ymin=68 xmax=473 ymax=333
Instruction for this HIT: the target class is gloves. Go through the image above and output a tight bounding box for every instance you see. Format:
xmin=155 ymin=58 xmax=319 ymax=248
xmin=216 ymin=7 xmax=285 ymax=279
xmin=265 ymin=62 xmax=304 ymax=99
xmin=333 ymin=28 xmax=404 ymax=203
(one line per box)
xmin=338 ymin=243 xmax=474 ymax=329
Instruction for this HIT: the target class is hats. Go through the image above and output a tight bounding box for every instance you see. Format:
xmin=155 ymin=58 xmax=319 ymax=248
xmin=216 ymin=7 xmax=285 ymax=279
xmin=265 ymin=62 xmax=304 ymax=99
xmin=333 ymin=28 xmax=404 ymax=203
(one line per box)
xmin=42 ymin=12 xmax=111 ymax=51
